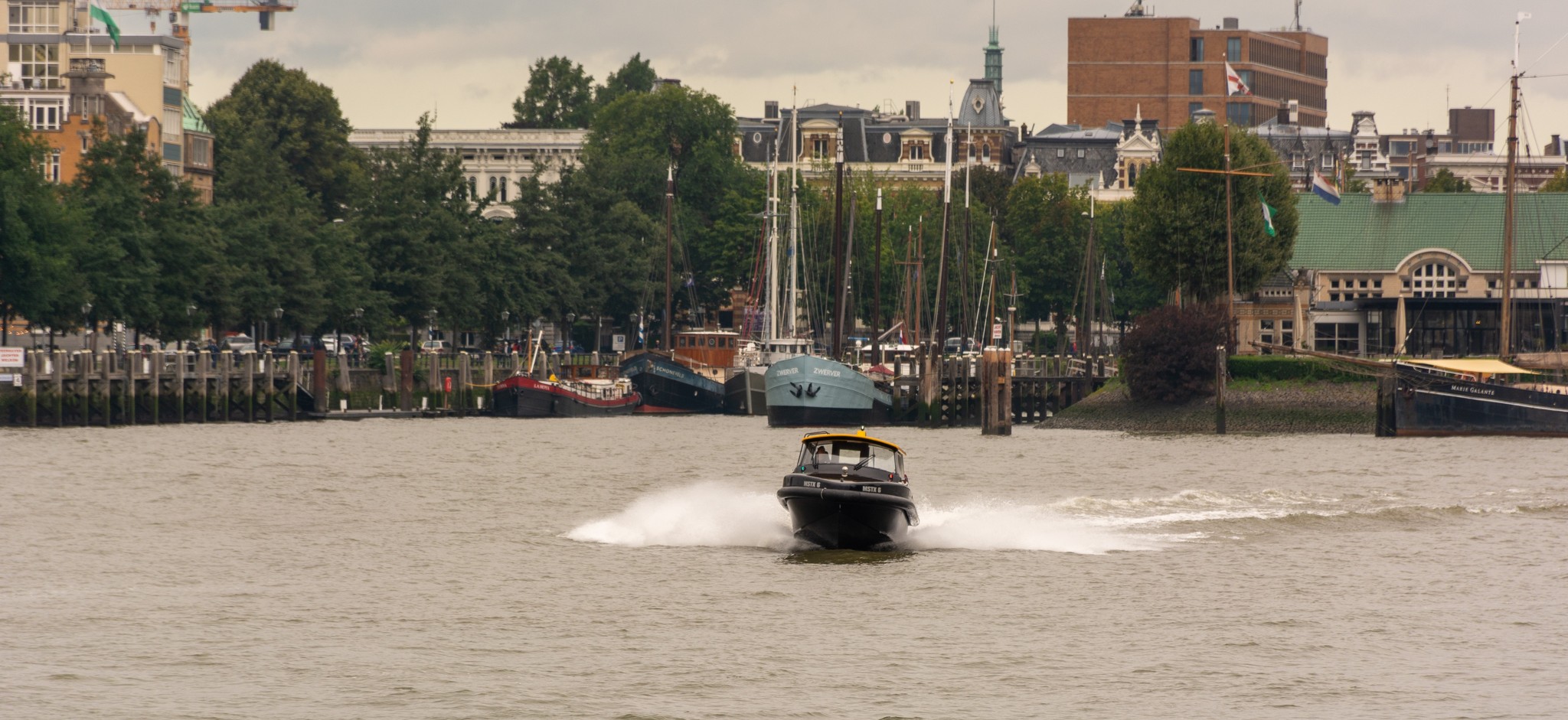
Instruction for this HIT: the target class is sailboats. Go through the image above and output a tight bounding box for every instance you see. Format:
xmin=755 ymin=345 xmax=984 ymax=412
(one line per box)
xmin=1382 ymin=19 xmax=1567 ymax=436
xmin=608 ymin=84 xmax=1129 ymax=379
xmin=618 ymin=135 xmax=727 ymax=415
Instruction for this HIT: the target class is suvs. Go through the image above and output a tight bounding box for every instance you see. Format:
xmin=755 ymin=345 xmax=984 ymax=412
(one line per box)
xmin=552 ymin=339 xmax=584 ymax=354
xmin=493 ymin=338 xmax=551 ymax=354
xmin=936 ymin=336 xmax=979 ymax=354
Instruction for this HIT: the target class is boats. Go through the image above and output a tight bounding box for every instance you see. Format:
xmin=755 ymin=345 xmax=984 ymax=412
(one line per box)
xmin=765 ymin=356 xmax=893 ymax=428
xmin=776 ymin=427 xmax=921 ymax=552
xmin=490 ymin=327 xmax=642 ymax=417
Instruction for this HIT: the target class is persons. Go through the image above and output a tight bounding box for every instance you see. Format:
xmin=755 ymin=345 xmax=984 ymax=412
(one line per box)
xmin=349 ymin=334 xmax=363 ymax=361
xmin=653 ymin=340 xmax=662 ymax=357
xmin=816 ymin=446 xmax=828 ymax=464
xmin=221 ymin=339 xmax=231 ymax=357
xmin=495 ymin=341 xmax=522 ymax=368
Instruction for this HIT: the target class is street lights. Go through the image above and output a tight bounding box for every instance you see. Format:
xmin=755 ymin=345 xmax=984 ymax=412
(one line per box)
xmin=274 ymin=305 xmax=284 ymax=344
xmin=428 ymin=306 xmax=438 ymax=352
xmin=688 ymin=311 xmax=697 ymax=331
xmin=187 ymin=301 xmax=198 ymax=342
xmin=80 ymin=298 xmax=93 ymax=350
xmin=629 ymin=310 xmax=638 ymax=351
xmin=355 ymin=306 xmax=364 ymax=354
xmin=501 ymin=307 xmax=510 ymax=354
xmin=567 ymin=310 xmax=576 ymax=353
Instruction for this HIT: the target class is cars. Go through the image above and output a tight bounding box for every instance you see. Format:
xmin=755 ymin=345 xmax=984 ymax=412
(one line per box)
xmin=421 ymin=341 xmax=454 ymax=355
xmin=457 ymin=346 xmax=486 ymax=356
xmin=22 ymin=334 xmax=371 ymax=360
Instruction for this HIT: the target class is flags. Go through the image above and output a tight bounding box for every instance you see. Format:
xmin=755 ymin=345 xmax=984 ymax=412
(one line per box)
xmin=899 ymin=325 xmax=907 ymax=345
